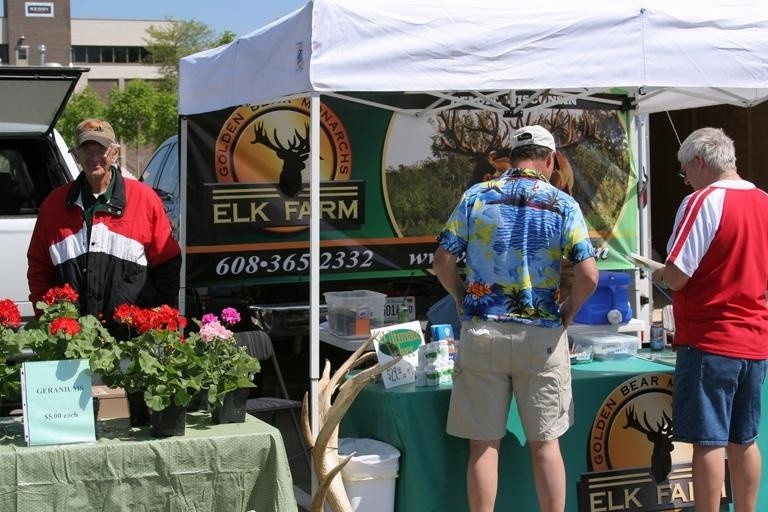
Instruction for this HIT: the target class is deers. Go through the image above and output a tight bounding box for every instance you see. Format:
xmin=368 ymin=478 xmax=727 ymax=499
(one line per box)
xmin=509 ymin=108 xmax=581 ymax=195
xmin=429 ymin=107 xmax=509 ymax=188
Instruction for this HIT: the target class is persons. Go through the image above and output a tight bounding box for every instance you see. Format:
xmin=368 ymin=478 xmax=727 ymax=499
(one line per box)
xmin=652 ymin=127 xmax=768 ymax=512
xmin=433 ymin=125 xmax=599 ymax=512
xmin=27 ymin=119 xmax=182 ymax=343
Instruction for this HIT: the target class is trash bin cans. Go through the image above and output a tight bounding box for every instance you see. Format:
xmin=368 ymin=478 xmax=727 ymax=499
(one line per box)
xmin=338 ymin=438 xmax=401 ymax=512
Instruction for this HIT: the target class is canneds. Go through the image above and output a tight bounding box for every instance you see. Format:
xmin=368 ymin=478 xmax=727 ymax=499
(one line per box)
xmin=650 ymin=323 xmax=665 ymax=351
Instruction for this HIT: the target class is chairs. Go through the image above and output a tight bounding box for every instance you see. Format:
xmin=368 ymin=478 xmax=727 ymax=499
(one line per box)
xmin=218 ymin=325 xmax=312 ymax=472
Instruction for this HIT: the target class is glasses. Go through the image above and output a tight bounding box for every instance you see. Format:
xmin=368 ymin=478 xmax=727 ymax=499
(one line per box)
xmin=676 ymin=166 xmax=686 ymax=178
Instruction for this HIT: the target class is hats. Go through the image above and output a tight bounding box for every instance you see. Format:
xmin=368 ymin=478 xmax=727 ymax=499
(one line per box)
xmin=73 ymin=119 xmax=115 ymax=148
xmin=510 ymin=124 xmax=560 ymax=170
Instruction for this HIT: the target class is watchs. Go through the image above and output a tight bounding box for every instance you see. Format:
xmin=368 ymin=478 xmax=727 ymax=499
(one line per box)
xmin=660 ymin=276 xmax=669 ymax=290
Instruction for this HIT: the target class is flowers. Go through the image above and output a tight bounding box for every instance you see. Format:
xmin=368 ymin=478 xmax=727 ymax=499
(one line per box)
xmin=1 ymin=287 xmax=263 ymax=440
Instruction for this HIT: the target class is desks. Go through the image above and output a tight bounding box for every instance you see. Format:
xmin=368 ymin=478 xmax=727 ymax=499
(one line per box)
xmin=314 ymin=323 xmax=382 ymax=359
xmin=0 ymin=408 xmax=300 ymax=511
xmin=329 ymin=349 xmax=767 ymax=512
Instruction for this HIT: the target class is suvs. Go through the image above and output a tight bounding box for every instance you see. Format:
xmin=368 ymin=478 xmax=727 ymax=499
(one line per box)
xmin=0 ymin=63 xmax=92 ymax=326
xmin=131 ymin=130 xmax=442 ymax=339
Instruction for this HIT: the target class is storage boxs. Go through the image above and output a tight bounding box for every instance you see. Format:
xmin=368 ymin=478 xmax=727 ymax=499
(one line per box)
xmin=315 ymin=288 xmax=388 ymax=340
xmin=88 ymin=380 xmax=130 ymax=423
xmin=573 ymin=330 xmax=642 ymax=362
